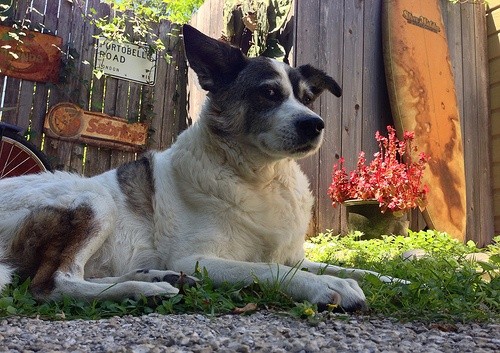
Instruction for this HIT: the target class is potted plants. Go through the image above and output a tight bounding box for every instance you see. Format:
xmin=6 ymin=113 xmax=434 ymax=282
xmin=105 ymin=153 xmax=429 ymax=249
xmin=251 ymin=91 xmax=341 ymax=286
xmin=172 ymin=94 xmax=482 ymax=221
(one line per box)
xmin=329 ymin=125 xmax=434 ymax=242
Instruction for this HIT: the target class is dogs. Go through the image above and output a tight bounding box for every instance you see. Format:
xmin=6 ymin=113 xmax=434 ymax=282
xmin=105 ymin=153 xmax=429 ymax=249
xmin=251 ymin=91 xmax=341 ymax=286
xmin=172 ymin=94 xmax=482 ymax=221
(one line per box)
xmin=0 ymin=17 xmax=414 ymax=312
